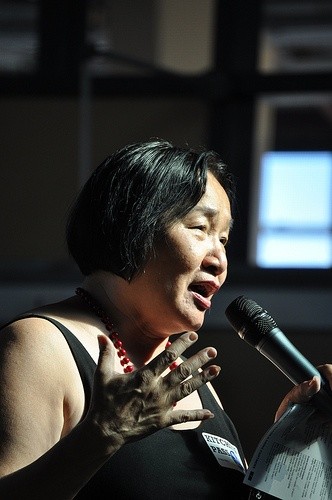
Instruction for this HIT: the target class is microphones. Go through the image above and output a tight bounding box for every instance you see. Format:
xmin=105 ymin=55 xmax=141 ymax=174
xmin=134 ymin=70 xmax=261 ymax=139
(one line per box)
xmin=224 ymin=296 xmax=327 ymax=396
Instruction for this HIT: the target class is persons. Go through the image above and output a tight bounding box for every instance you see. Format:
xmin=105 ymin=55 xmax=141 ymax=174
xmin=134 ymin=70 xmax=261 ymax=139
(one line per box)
xmin=0 ymin=138 xmax=332 ymax=500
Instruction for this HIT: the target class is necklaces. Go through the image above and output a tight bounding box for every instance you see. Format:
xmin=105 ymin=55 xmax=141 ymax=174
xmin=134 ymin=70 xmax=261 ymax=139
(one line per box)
xmin=75 ymin=285 xmax=178 ymax=412
